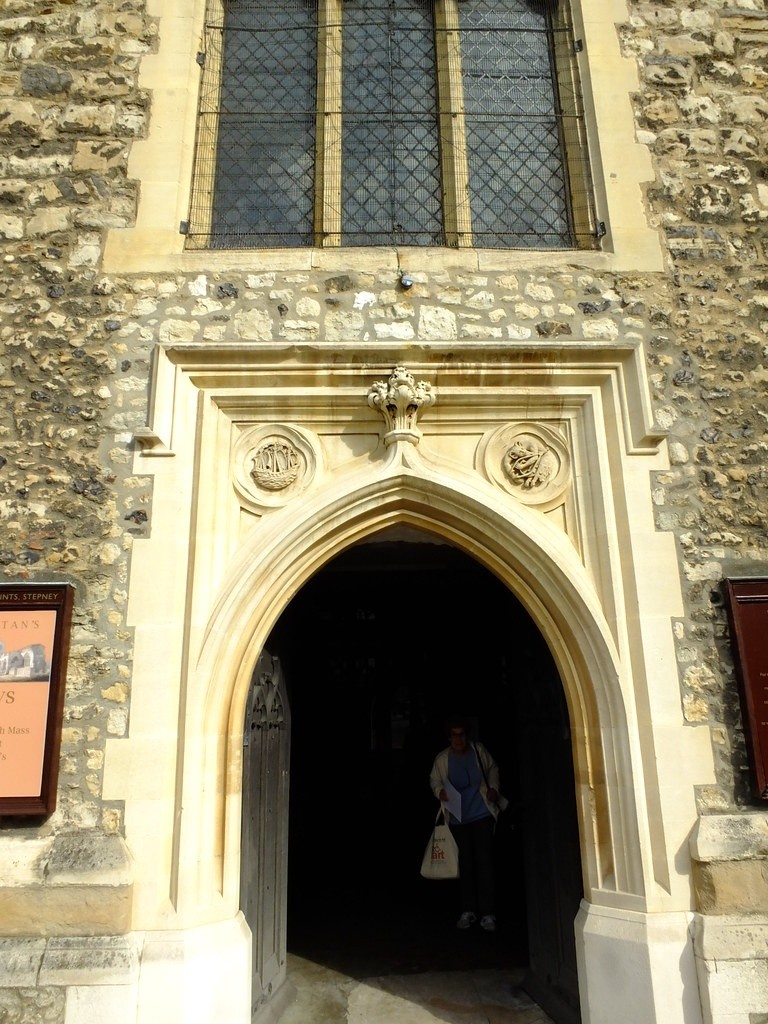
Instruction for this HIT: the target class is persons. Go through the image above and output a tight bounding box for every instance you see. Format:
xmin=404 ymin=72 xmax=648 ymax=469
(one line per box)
xmin=429 ymin=718 xmax=501 ymax=931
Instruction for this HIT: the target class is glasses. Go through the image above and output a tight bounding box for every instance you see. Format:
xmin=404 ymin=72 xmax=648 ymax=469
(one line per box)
xmin=449 ymin=732 xmax=465 ymax=738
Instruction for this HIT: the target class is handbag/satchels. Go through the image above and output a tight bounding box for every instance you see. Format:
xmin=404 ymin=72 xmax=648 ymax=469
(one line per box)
xmin=498 ymin=813 xmax=515 ymax=838
xmin=419 ymin=822 xmax=461 ymax=880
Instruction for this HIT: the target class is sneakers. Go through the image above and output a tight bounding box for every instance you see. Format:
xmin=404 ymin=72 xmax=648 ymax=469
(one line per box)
xmin=478 ymin=914 xmax=496 ymax=931
xmin=457 ymin=911 xmax=475 ymax=929
